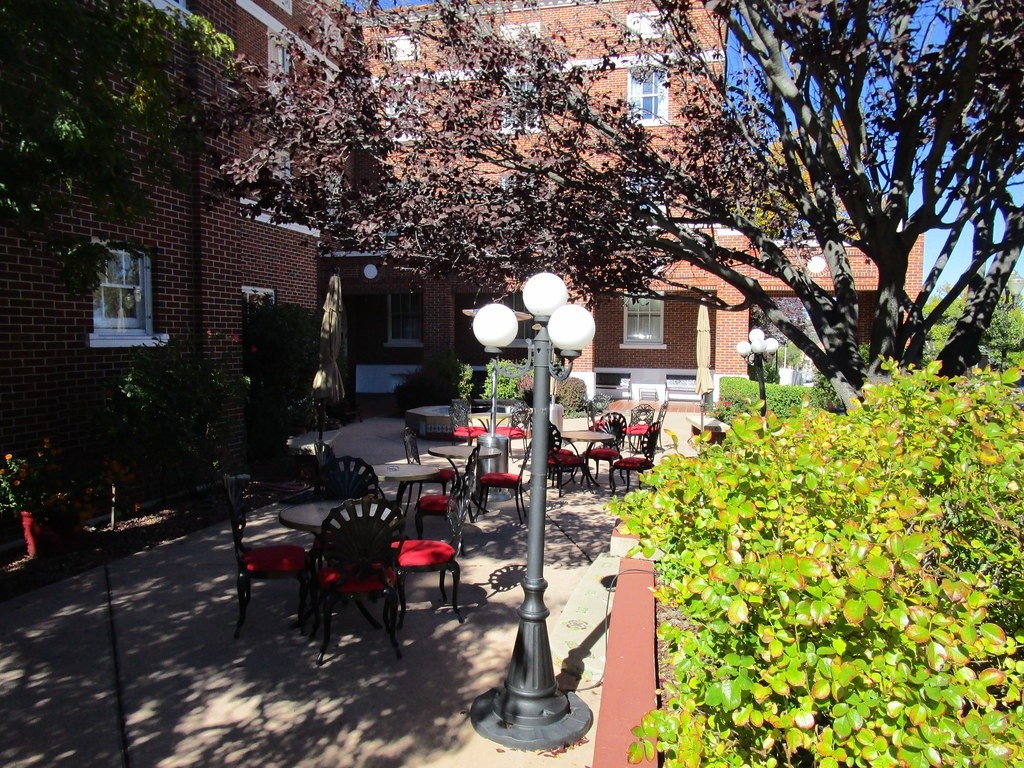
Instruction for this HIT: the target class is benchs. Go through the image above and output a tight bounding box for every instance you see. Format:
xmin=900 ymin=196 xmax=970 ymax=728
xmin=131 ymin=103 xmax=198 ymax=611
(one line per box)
xmin=686 ymin=416 xmax=730 ymax=445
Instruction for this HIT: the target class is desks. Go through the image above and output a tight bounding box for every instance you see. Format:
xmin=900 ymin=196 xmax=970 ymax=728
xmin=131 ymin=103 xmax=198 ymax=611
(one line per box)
xmin=559 ymin=430 xmax=617 ymax=488
xmin=278 ymin=500 xmax=405 ymax=628
xmin=427 ymin=444 xmax=502 ymax=523
xmin=366 ymin=463 xmax=440 ymax=516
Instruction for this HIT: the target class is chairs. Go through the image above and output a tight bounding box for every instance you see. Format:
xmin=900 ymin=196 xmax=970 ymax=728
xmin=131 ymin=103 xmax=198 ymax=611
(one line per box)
xmin=313 ymin=438 xmax=386 ymax=499
xmin=403 ymin=427 xmax=458 ymax=520
xmin=225 ymin=487 xmax=312 ymax=639
xmin=494 ymin=401 xmax=531 ymax=462
xmin=448 ymin=401 xmax=488 ymax=445
xmin=415 ymin=443 xmax=482 ymax=556
xmin=474 ymin=439 xmax=532 ymax=524
xmin=309 ymin=495 xmax=409 ymax=665
xmin=313 ymin=455 xmax=380 ymax=571
xmin=546 ymin=397 xmax=670 ymax=497
xmin=390 ymin=471 xmax=474 ymax=630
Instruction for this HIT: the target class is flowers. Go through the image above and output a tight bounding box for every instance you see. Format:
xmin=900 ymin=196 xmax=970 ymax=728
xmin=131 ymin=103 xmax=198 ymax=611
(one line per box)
xmin=0 ymin=435 xmax=143 ymax=535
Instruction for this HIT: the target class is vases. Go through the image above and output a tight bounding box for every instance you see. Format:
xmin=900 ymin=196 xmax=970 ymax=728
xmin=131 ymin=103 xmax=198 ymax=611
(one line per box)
xmin=19 ymin=510 xmax=62 ymax=556
